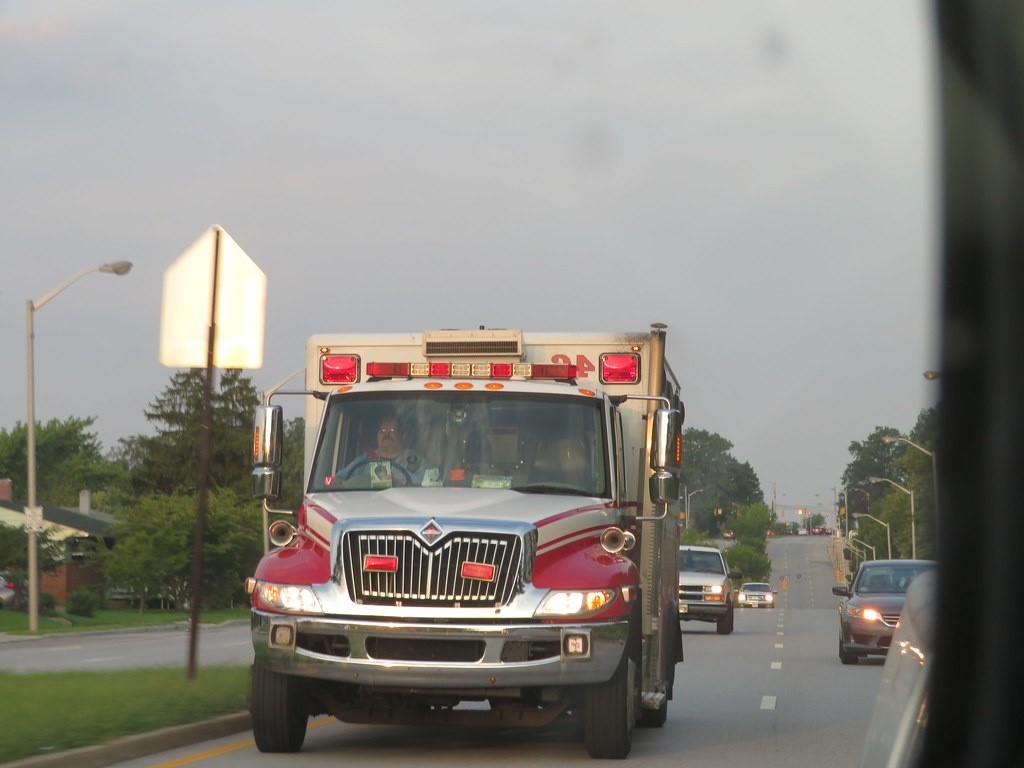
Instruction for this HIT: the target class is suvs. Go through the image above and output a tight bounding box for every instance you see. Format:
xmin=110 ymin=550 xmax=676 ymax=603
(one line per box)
xmin=675 ymin=545 xmax=741 ymax=634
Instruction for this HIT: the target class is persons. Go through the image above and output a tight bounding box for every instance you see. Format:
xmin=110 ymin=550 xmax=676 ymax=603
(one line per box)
xmin=332 ymin=414 xmax=428 ymax=489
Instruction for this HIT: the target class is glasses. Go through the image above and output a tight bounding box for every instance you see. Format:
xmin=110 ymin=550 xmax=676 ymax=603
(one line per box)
xmin=378 ymin=427 xmax=397 ymax=433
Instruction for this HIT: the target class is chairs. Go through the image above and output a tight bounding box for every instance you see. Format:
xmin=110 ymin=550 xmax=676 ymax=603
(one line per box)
xmin=365 ymin=415 xmax=441 ymax=497
xmin=527 ymin=436 xmax=594 ymax=493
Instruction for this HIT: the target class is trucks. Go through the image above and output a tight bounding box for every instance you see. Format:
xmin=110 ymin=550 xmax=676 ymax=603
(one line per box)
xmin=241 ymin=323 xmax=685 ymax=759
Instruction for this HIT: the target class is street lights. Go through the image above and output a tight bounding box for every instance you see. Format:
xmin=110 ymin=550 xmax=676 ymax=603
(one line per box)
xmin=882 ymin=436 xmax=940 ymax=560
xmin=839 ymin=489 xmax=849 ymax=581
xmin=25 ymin=261 xmax=136 ymax=630
xmin=869 ymin=477 xmax=916 ymax=560
xmin=686 ymin=489 xmax=705 ymax=526
xmin=853 ymin=513 xmax=891 ymax=560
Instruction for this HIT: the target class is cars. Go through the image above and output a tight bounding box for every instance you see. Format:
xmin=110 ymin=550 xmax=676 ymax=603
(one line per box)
xmin=0 ymin=576 xmax=17 ymax=610
xmin=786 ymin=526 xmax=835 ymax=537
xmin=735 ymin=582 xmax=778 ymax=608
xmin=832 ymin=559 xmax=942 ymax=665
